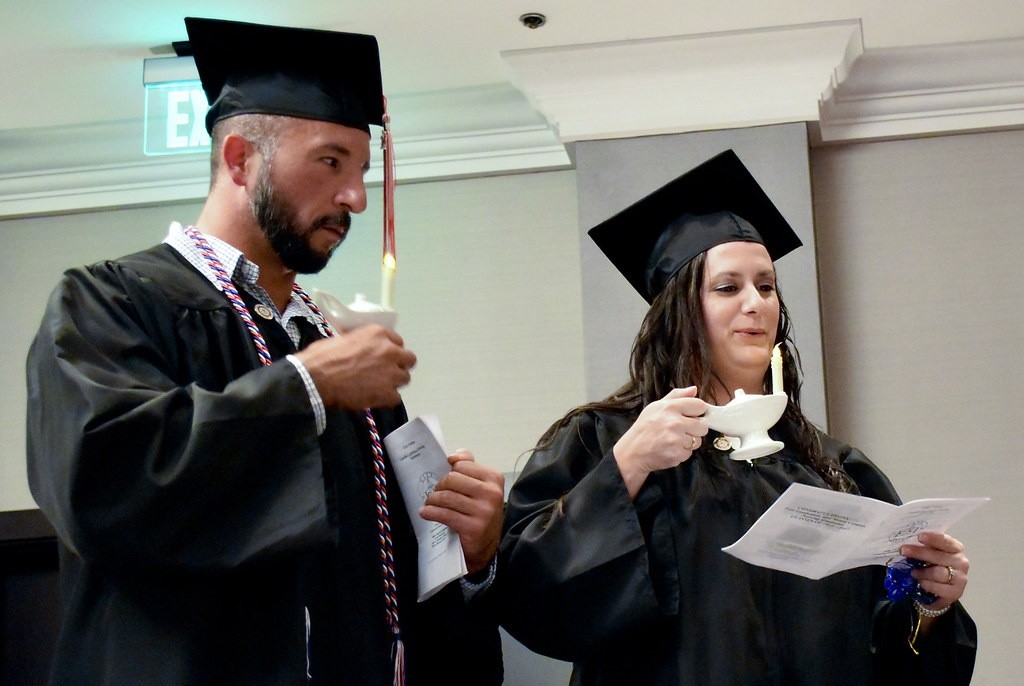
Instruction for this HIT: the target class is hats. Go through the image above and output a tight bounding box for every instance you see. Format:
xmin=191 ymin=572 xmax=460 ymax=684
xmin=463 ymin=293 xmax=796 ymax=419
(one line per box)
xmin=171 ymin=16 xmax=398 ymax=277
xmin=586 ymin=149 xmax=803 ymax=306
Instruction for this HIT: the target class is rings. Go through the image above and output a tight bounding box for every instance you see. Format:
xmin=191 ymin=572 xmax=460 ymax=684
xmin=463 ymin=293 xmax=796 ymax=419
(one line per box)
xmin=945 ymin=565 xmax=956 ymax=584
xmin=687 ymin=435 xmax=696 ymax=450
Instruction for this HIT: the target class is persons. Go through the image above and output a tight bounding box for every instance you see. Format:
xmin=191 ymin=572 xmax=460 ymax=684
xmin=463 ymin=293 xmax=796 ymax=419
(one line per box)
xmin=25 ymin=17 xmax=505 ymax=686
xmin=500 ymin=149 xmax=979 ymax=686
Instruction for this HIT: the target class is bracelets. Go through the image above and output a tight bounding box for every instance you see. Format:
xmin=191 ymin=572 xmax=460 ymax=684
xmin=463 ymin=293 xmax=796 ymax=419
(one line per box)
xmin=911 ymin=597 xmax=951 ymax=618
